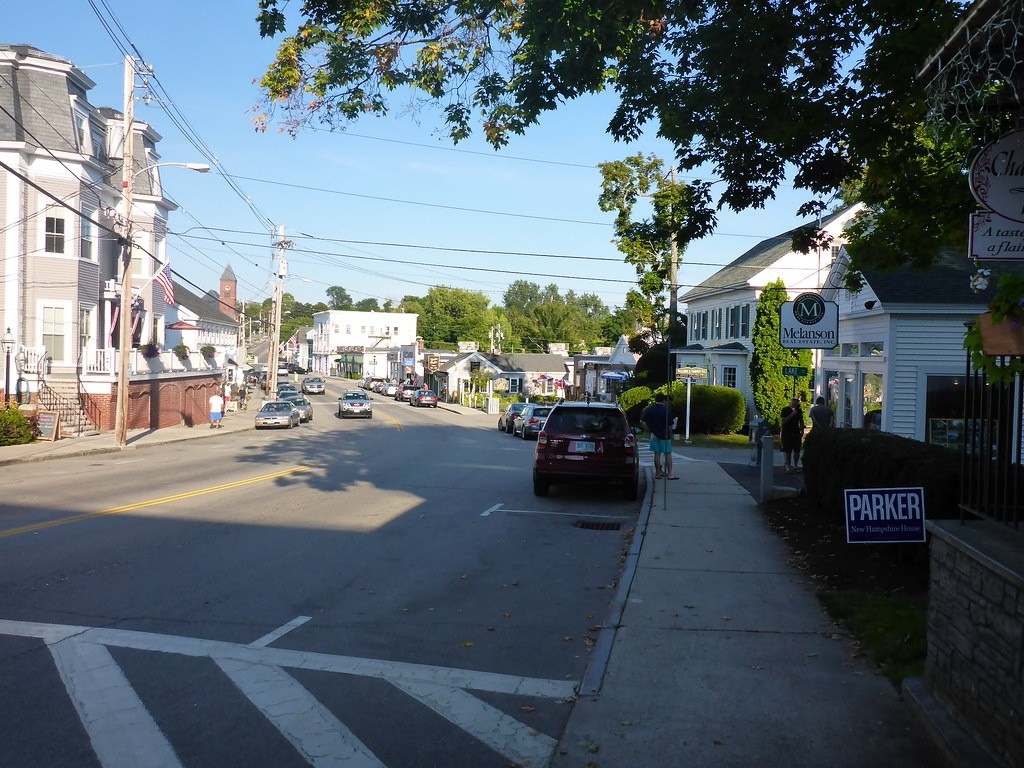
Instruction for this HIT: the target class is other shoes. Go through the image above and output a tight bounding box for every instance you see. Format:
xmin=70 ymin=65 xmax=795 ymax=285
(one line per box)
xmin=218 ymin=424 xmax=221 ymax=428
xmin=785 ymin=464 xmax=792 ymax=474
xmin=793 ymin=467 xmax=801 ymax=474
xmin=210 ymin=423 xmax=213 ymax=429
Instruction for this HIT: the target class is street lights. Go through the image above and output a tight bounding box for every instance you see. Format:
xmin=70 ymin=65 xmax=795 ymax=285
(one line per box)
xmin=114 ymin=161 xmax=213 ymax=447
xmin=266 ymin=276 xmax=312 ymax=401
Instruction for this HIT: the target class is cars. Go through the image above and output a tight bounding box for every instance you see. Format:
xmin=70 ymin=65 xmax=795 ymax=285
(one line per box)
xmin=409 ymin=389 xmax=438 ymax=408
xmin=254 ymin=362 xmax=313 ymax=423
xmin=513 ymin=404 xmax=553 ymax=441
xmin=338 ymin=388 xmax=374 ymax=420
xmin=301 ymin=376 xmax=326 ymax=395
xmin=497 ymin=402 xmax=531 ymax=434
xmin=254 ymin=401 xmax=300 ymax=430
xmin=358 ymin=375 xmax=420 ymax=402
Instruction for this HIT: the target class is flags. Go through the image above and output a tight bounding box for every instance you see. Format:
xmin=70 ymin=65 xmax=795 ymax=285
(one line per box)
xmin=153 ymin=259 xmax=174 ymax=307
xmin=413 ymin=373 xmax=421 ymax=386
xmin=280 ymin=332 xmax=297 ymax=352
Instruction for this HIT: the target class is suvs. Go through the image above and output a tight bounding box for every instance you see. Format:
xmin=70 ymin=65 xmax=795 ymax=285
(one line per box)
xmin=531 ymin=397 xmax=640 ymax=499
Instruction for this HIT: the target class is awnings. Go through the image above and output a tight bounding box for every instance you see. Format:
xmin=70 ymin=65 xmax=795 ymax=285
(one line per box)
xmin=603 ymin=371 xmax=632 ymax=380
xmin=534 ymin=374 xmax=554 ymax=384
xmin=554 ymin=378 xmax=574 ymax=397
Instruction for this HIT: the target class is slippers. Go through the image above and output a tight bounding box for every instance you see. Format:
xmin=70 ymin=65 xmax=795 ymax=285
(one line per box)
xmin=655 ymin=474 xmax=661 ymax=479
xmin=668 ymin=475 xmax=679 ymax=480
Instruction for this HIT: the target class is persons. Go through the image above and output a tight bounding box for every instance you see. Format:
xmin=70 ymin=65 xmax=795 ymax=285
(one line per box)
xmin=253 ymin=376 xmax=257 ymax=389
xmin=640 ymin=392 xmax=678 ymax=480
xmin=386 ymin=378 xmax=429 ymax=390
xmin=209 ymin=390 xmax=224 ymax=429
xmin=225 ymin=383 xmax=232 ymax=403
xmin=238 ymin=387 xmax=247 ymax=410
xmin=810 ymin=397 xmax=834 ymax=431
xmin=780 ymin=398 xmax=804 ymax=474
